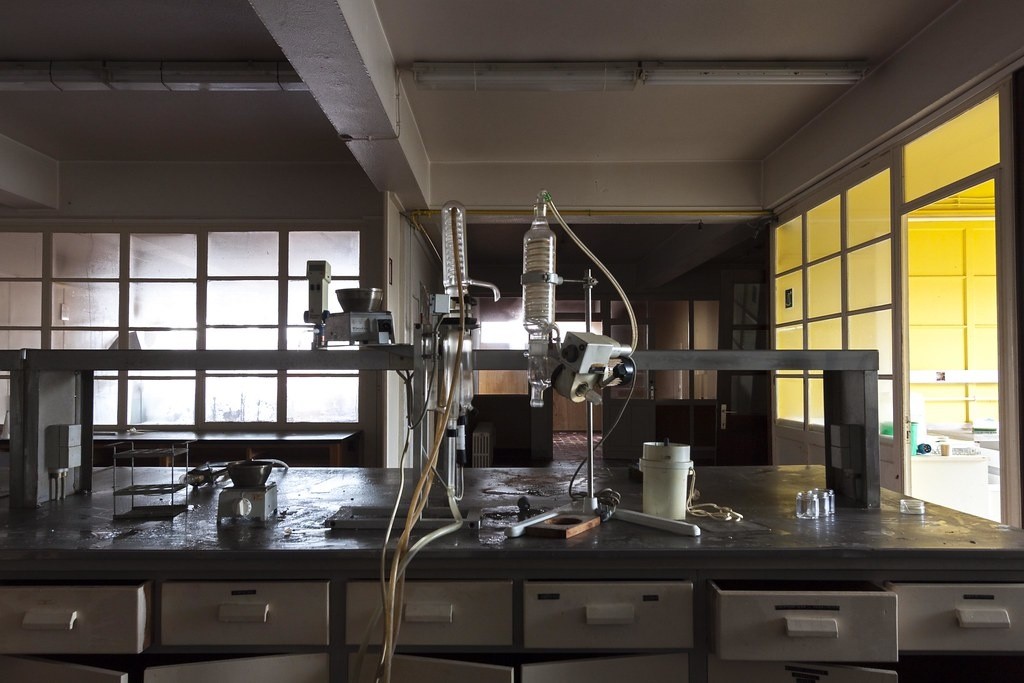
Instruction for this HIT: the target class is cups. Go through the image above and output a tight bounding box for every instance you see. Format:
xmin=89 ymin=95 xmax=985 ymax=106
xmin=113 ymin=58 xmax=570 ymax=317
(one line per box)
xmin=940 ymin=444 xmax=950 ymax=455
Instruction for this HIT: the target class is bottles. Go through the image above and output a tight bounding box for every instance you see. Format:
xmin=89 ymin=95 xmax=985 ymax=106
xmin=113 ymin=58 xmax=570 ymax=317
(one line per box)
xmin=795 ymin=488 xmax=835 ymax=519
